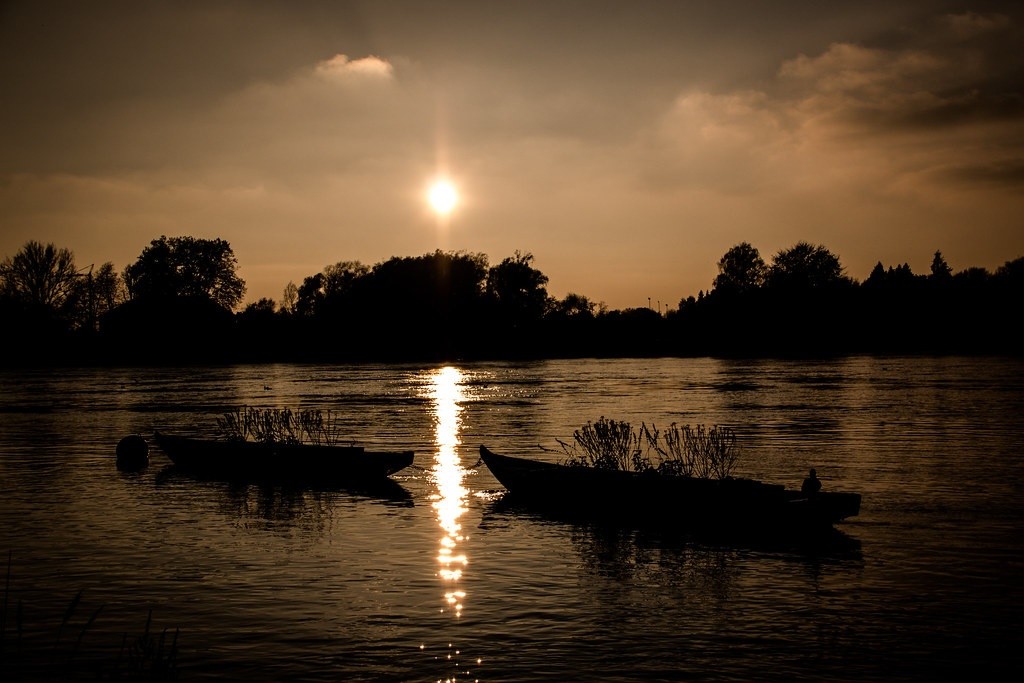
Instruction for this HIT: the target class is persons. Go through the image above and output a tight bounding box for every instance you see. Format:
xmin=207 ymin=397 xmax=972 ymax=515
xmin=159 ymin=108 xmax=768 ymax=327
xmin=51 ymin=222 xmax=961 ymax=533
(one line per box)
xmin=801 ymin=470 xmax=822 ymax=492
